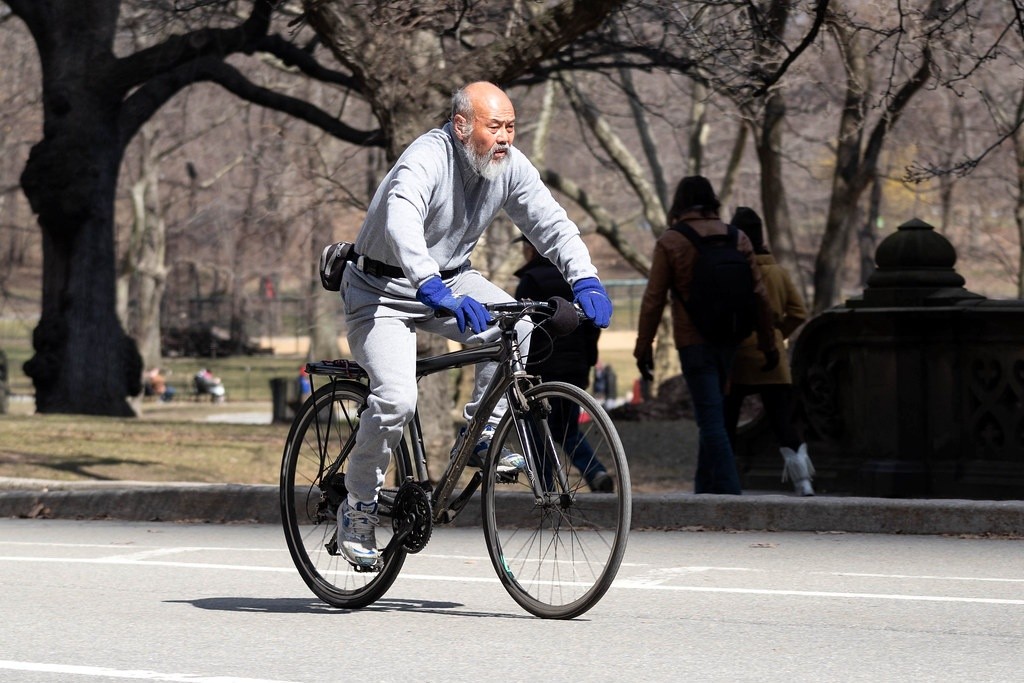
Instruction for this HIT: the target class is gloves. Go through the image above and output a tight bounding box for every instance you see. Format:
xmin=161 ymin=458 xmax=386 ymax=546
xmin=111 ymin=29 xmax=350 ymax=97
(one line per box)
xmin=573 ymin=277 xmax=613 ymax=326
xmin=417 ymin=276 xmax=491 ymax=334
xmin=637 ymin=355 xmax=654 ymax=381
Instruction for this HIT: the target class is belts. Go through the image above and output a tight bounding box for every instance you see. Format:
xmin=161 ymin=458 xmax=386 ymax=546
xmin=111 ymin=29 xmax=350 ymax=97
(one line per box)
xmin=349 ymin=251 xmax=460 ymax=280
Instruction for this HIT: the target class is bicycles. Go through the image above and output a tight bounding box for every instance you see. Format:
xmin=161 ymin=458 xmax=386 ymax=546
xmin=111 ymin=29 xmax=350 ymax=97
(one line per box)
xmin=278 ymin=298 xmax=632 ymax=620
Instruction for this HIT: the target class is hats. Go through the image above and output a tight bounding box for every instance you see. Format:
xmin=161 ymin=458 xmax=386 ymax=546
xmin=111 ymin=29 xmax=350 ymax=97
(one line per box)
xmin=511 ymin=224 xmax=530 ymax=244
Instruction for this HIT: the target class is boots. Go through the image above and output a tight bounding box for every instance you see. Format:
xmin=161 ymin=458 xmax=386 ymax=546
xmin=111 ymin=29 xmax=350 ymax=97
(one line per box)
xmin=779 ymin=442 xmax=816 ymax=496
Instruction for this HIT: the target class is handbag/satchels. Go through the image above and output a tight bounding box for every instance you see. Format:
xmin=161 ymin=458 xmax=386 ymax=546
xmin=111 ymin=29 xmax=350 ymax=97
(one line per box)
xmin=319 ymin=241 xmax=354 ymax=292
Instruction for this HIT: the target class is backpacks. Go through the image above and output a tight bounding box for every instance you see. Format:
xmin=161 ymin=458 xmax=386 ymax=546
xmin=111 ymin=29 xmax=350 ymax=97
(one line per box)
xmin=670 ymin=221 xmax=756 ymax=344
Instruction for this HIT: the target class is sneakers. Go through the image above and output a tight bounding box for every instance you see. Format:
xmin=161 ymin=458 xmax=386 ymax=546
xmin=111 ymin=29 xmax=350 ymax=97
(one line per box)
xmin=449 ymin=425 xmax=526 ymax=474
xmin=336 ymin=497 xmax=380 ymax=566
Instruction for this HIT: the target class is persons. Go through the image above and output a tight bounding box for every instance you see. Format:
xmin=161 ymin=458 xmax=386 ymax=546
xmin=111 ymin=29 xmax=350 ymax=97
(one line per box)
xmin=146 ymin=365 xmax=226 ymax=404
xmin=633 ymin=176 xmax=815 ymax=495
xmin=509 ymin=234 xmax=616 ymax=493
xmin=337 ymin=81 xmax=614 ymax=567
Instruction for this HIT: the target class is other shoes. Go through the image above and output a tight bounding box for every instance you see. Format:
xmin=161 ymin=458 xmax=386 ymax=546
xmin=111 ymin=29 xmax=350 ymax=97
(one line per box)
xmin=592 ymin=471 xmax=615 ymax=494
